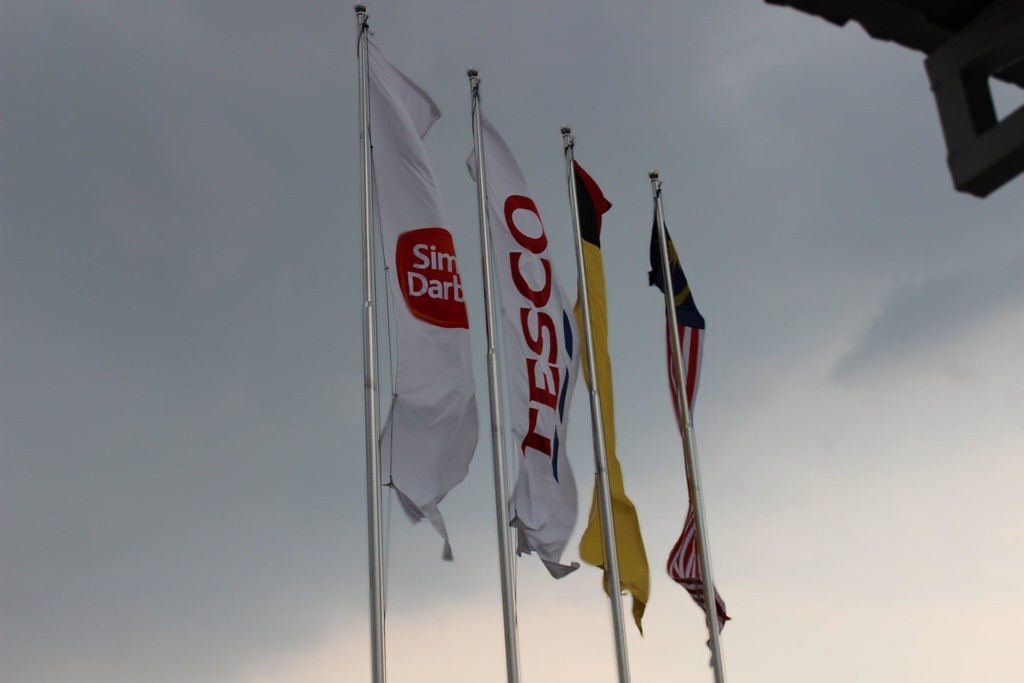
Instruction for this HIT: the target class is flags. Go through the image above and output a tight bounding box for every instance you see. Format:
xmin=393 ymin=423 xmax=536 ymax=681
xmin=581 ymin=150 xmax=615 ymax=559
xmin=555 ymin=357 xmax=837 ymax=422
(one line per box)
xmin=573 ymin=160 xmax=649 ymax=637
xmin=365 ymin=41 xmax=480 ymax=561
xmin=473 ymin=114 xmax=580 ymax=578
xmin=648 ymin=221 xmax=730 ymax=661
